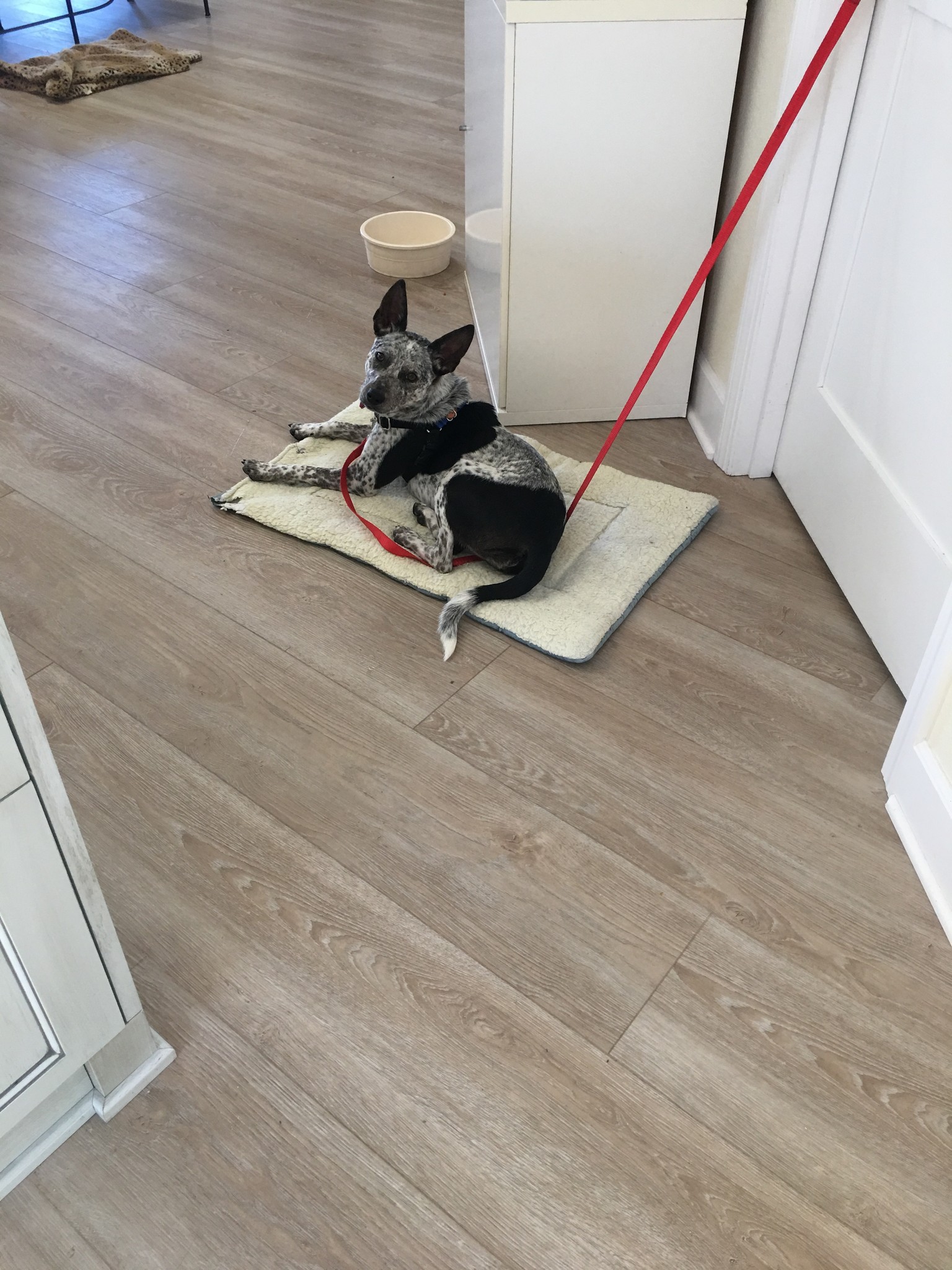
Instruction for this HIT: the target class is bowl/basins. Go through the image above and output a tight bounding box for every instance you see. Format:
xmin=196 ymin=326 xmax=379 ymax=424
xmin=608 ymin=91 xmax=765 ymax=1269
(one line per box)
xmin=360 ymin=211 xmax=455 ymax=279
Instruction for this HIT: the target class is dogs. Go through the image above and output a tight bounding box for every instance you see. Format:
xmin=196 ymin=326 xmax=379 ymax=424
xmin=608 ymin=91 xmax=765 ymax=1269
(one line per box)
xmin=241 ymin=278 xmax=572 ymax=662
